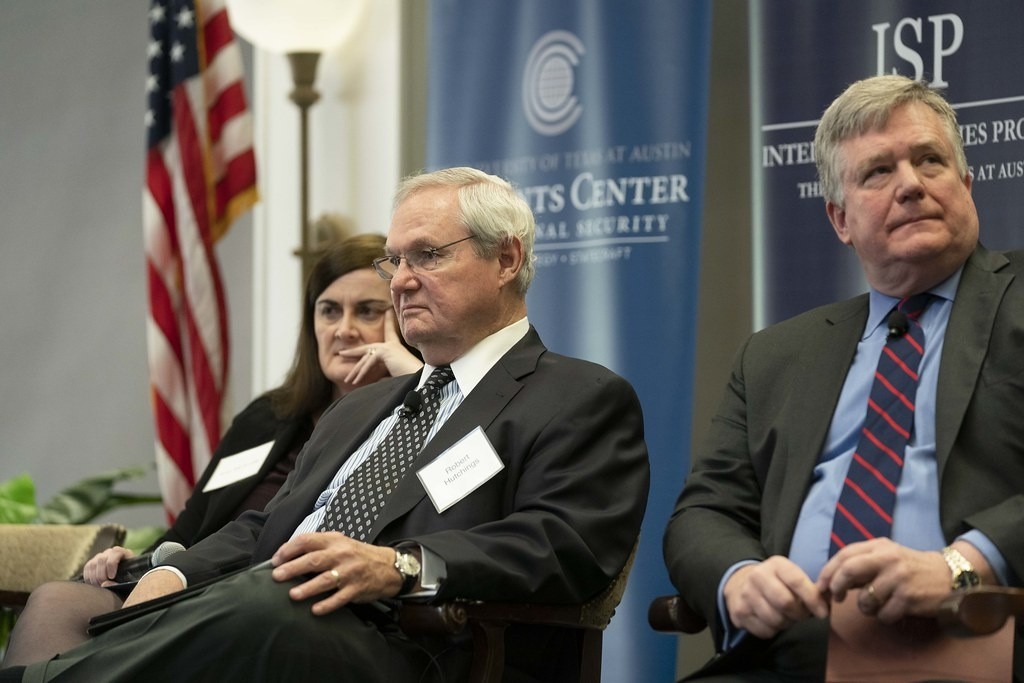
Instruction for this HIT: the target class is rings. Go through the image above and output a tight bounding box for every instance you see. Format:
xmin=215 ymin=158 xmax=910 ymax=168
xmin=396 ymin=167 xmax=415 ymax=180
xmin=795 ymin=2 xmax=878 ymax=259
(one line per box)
xmin=866 ymin=583 xmax=884 ymax=610
xmin=370 ymin=349 xmax=375 ymax=356
xmin=332 ymin=569 xmax=342 ymax=590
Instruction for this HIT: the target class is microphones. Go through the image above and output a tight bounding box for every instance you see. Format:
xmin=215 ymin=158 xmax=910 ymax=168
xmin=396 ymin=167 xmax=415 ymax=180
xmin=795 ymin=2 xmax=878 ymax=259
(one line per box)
xmin=69 ymin=541 xmax=187 ymax=585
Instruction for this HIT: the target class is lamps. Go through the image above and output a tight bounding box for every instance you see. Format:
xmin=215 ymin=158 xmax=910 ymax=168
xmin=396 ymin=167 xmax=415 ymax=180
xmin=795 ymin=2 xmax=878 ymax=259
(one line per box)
xmin=222 ymin=0 xmax=368 ymax=298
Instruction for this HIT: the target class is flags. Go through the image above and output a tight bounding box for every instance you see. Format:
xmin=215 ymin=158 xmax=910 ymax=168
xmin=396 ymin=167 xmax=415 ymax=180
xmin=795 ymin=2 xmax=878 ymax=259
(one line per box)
xmin=148 ymin=2 xmax=264 ymax=532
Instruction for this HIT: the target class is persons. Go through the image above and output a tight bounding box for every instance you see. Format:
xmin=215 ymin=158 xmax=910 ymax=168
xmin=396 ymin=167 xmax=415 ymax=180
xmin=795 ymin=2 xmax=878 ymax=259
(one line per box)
xmin=1 ymin=165 xmax=652 ymax=683
xmin=664 ymin=75 xmax=1024 ymax=683
xmin=2 ymin=236 xmax=426 ymax=675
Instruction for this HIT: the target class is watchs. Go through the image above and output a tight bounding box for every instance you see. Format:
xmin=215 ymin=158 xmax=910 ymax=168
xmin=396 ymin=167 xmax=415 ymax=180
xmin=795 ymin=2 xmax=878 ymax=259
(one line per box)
xmin=943 ymin=547 xmax=984 ymax=593
xmin=394 ymin=547 xmax=423 ymax=603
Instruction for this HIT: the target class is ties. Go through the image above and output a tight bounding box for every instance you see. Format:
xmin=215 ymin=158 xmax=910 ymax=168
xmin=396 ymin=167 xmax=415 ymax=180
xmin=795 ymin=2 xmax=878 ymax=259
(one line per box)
xmin=829 ymin=293 xmax=927 ymax=560
xmin=316 ymin=365 xmax=456 ymax=542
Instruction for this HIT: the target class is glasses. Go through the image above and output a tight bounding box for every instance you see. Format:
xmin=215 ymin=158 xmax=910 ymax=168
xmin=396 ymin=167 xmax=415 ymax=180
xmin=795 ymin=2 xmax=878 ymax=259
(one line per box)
xmin=371 ymin=234 xmax=478 ymax=282
